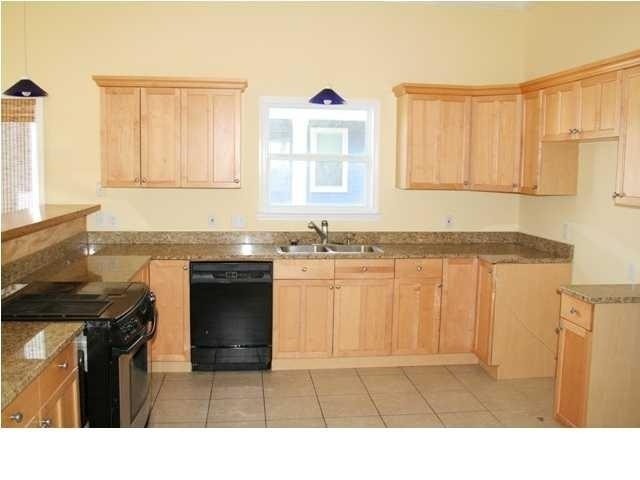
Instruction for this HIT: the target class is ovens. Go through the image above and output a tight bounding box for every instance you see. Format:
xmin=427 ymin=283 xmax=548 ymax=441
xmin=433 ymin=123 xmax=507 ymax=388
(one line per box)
xmin=109 ymin=337 xmax=153 ymax=427
xmin=189 ymin=262 xmax=273 ymax=346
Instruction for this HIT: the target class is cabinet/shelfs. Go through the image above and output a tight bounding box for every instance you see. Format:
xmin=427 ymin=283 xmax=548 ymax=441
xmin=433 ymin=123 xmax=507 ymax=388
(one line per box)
xmin=394 ymin=48 xmax=640 ymax=207
xmin=130 ymin=260 xmax=193 ymax=374
xmin=92 ymin=74 xmax=249 ymax=190
xmin=550 ymin=291 xmax=640 ymax=428
xmin=1 ymin=333 xmax=85 ymax=428
xmin=271 ymin=259 xmax=495 ymax=371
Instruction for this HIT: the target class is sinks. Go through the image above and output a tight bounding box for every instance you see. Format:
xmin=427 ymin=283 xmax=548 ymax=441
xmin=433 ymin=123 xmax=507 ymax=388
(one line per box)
xmin=273 ymin=243 xmax=329 ymax=257
xmin=329 ymin=243 xmax=385 ymax=255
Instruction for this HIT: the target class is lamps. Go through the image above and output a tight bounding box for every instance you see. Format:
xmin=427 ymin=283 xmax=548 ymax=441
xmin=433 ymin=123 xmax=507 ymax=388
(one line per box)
xmin=310 ymin=85 xmax=347 ymax=105
xmin=1 ymin=2 xmax=48 ymax=98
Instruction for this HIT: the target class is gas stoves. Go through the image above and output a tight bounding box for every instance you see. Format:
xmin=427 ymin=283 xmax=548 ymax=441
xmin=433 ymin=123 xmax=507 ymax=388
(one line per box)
xmin=1 ymin=280 xmax=147 ymax=320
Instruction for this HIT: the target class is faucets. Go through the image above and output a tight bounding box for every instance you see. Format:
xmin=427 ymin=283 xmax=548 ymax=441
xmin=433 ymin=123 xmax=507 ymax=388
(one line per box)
xmin=308 ymin=219 xmax=329 ymax=245
